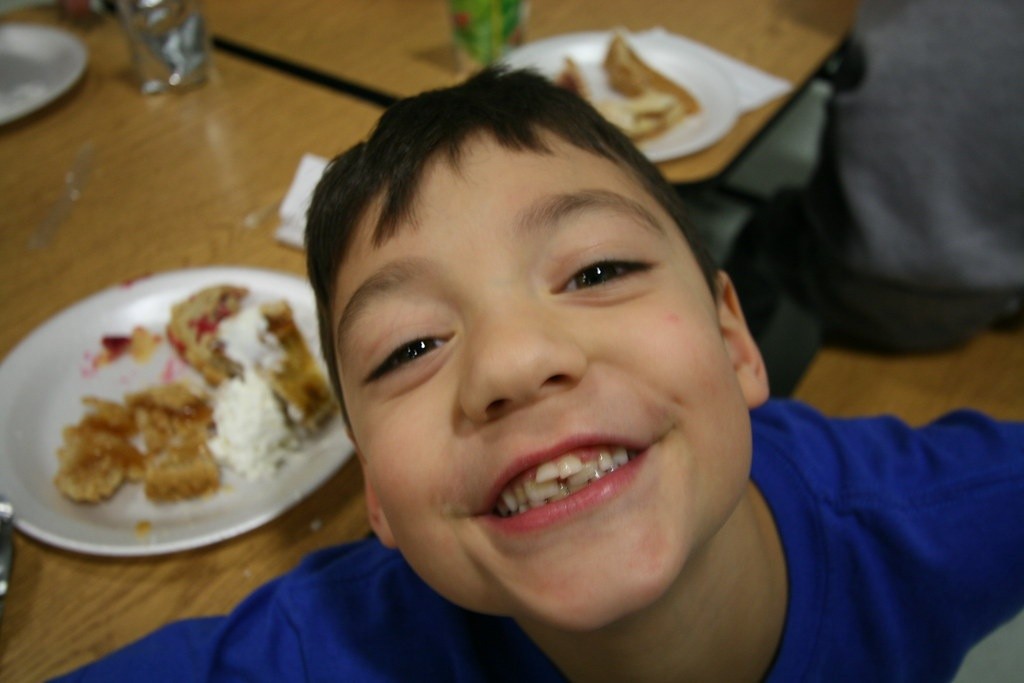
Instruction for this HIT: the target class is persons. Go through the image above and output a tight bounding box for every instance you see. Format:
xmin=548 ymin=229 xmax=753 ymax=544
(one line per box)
xmin=47 ymin=61 xmax=1024 ymax=683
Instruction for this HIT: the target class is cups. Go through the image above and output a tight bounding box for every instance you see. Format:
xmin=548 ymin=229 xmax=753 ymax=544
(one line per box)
xmin=117 ymin=0 xmax=209 ymax=95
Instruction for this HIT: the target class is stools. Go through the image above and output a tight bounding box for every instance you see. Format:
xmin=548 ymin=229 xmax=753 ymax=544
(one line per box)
xmin=792 ymin=328 xmax=1024 ymax=426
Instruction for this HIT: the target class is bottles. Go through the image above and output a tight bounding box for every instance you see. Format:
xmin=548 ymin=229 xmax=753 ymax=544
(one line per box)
xmin=449 ymin=0 xmax=531 ymax=73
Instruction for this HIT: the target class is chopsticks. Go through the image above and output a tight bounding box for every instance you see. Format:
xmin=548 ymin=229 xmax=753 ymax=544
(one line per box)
xmin=210 ymin=33 xmax=400 ymax=109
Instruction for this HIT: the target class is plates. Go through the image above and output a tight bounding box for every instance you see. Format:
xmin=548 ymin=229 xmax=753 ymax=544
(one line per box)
xmin=498 ymin=30 xmax=740 ymax=164
xmin=0 ymin=266 xmax=356 ymax=557
xmin=0 ymin=25 xmax=88 ymax=127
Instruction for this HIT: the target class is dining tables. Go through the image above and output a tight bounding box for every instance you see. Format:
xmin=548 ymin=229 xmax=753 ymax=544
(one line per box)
xmin=0 ymin=0 xmax=853 ymax=683
